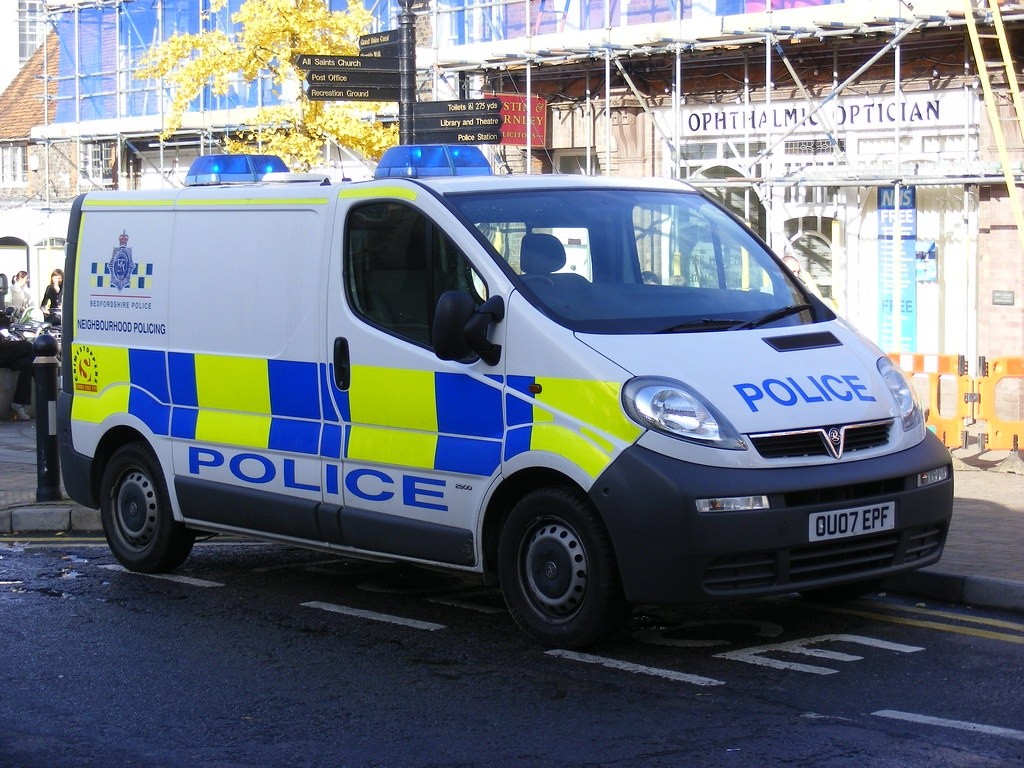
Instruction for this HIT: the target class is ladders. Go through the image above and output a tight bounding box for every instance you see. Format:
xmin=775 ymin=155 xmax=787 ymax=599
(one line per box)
xmin=962 ymin=0 xmax=1024 ymax=254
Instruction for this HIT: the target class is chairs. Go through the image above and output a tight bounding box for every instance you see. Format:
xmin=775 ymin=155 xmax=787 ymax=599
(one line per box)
xmin=518 ymin=233 xmax=591 ymax=285
xmin=355 ymin=226 xmax=447 ymax=345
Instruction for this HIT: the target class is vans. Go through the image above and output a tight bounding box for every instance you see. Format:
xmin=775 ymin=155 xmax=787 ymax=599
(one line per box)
xmin=58 ymin=145 xmax=957 ymax=649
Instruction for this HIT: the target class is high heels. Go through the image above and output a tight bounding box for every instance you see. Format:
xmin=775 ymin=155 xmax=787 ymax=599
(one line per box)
xmin=11 ymin=402 xmax=30 ymax=420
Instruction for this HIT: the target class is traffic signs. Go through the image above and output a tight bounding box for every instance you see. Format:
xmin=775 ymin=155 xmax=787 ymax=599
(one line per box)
xmin=297 ymin=27 xmax=504 ymax=146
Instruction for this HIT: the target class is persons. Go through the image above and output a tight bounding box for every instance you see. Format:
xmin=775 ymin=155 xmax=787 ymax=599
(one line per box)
xmin=39 ymin=268 xmax=64 ymax=326
xmin=781 ymin=255 xmax=801 ymax=278
xmin=0 ymin=309 xmax=61 ymax=420
xmin=9 ymin=270 xmax=32 ymax=315
